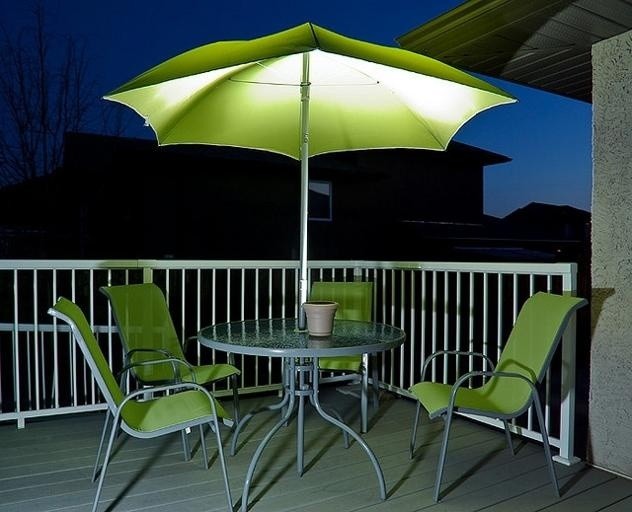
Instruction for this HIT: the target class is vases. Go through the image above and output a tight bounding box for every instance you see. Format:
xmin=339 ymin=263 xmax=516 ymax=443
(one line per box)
xmin=302 ymin=301 xmax=338 ymax=338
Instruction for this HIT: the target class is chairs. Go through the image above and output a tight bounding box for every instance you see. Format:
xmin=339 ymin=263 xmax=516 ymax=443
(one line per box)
xmin=48 ymin=296 xmax=234 ymax=512
xmin=100 ymin=283 xmax=242 ymax=461
xmin=409 ymin=292 xmax=589 ymax=503
xmin=281 ymin=281 xmax=379 ymax=434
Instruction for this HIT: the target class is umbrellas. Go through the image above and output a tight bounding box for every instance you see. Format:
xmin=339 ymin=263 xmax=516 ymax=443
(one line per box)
xmin=99 ymin=22 xmax=521 ymax=329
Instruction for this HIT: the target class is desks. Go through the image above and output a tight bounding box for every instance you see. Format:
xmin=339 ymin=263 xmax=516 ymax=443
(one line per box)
xmin=198 ymin=318 xmax=406 ymax=512
